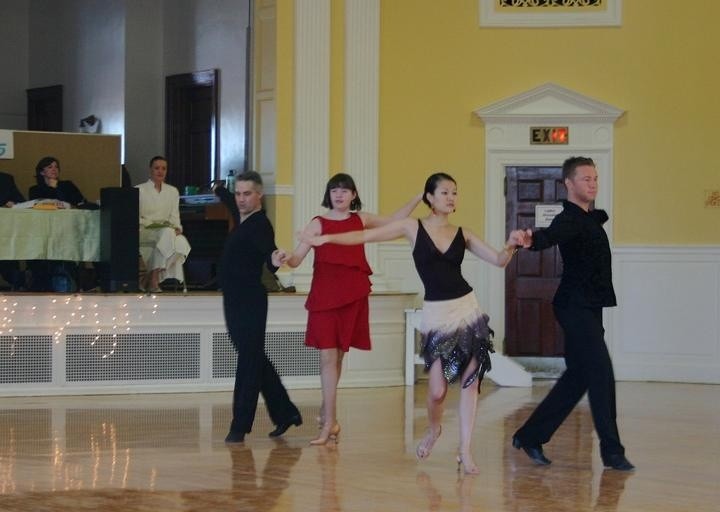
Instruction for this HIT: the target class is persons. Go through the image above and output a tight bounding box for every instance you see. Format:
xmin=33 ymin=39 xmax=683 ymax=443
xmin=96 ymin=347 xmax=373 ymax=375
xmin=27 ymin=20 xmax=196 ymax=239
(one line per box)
xmin=276 ymin=171 xmax=425 ymax=446
xmin=0 ymin=171 xmax=28 ymax=288
xmin=132 ymin=153 xmax=194 ymax=293
xmin=206 ymin=170 xmax=304 ymax=442
xmin=29 ymin=156 xmax=89 ymax=211
xmin=510 ymin=155 xmax=637 ymax=472
xmin=294 ymin=171 xmax=529 ymax=476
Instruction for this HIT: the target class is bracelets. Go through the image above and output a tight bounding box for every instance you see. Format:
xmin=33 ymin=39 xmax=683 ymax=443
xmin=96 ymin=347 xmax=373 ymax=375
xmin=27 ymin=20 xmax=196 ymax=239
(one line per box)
xmin=503 ymin=241 xmax=519 ymax=258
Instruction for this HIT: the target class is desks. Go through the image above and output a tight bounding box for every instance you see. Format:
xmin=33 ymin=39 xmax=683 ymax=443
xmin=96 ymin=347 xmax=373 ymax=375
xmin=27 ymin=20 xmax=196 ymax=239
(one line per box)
xmin=0 ymin=206 xmax=101 ymax=291
xmin=180 ymin=203 xmax=234 ymax=287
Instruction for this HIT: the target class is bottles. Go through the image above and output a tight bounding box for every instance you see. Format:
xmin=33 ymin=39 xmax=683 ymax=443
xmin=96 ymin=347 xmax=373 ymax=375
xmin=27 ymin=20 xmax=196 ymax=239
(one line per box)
xmin=225 ymin=170 xmax=236 ymax=192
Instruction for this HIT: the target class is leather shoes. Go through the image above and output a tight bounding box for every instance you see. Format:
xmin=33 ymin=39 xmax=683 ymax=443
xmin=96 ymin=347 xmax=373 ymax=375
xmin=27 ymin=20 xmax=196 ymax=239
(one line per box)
xmin=225 ymin=427 xmax=244 ymax=442
xmin=269 ymin=413 xmax=302 ymax=436
xmin=603 ymin=455 xmax=633 ymax=469
xmin=513 ymin=436 xmax=552 ymax=465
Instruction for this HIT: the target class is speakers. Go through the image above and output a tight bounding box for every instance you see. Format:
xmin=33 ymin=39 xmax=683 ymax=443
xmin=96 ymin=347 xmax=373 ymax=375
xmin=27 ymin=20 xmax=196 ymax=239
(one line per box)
xmin=100 ymin=187 xmax=139 ymax=291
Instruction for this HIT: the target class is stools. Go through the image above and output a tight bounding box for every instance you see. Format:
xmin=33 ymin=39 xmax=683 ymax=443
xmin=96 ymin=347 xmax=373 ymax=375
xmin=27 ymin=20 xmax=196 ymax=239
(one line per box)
xmin=404 ymin=308 xmax=425 ymax=386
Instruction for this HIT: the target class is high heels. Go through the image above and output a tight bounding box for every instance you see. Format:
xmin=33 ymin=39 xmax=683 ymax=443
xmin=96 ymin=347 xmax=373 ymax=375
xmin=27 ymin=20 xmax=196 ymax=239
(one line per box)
xmin=416 ymin=424 xmax=442 ymax=459
xmin=457 ymin=456 xmax=477 ymax=474
xmin=309 ymin=416 xmax=340 ymax=445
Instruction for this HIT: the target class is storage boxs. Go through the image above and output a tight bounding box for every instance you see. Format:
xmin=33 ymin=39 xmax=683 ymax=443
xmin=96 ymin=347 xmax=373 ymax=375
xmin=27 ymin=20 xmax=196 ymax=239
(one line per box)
xmin=188 ymin=257 xmax=215 ymax=283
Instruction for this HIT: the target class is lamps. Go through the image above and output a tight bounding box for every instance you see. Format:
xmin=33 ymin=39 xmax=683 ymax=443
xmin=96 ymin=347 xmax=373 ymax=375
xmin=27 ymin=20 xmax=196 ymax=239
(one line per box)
xmin=80 ymin=116 xmax=99 ymax=133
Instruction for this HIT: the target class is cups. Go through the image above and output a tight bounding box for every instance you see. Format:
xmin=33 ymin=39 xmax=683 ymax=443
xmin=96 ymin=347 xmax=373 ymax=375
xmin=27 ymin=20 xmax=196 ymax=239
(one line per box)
xmin=183 ymin=185 xmax=198 ymax=196
